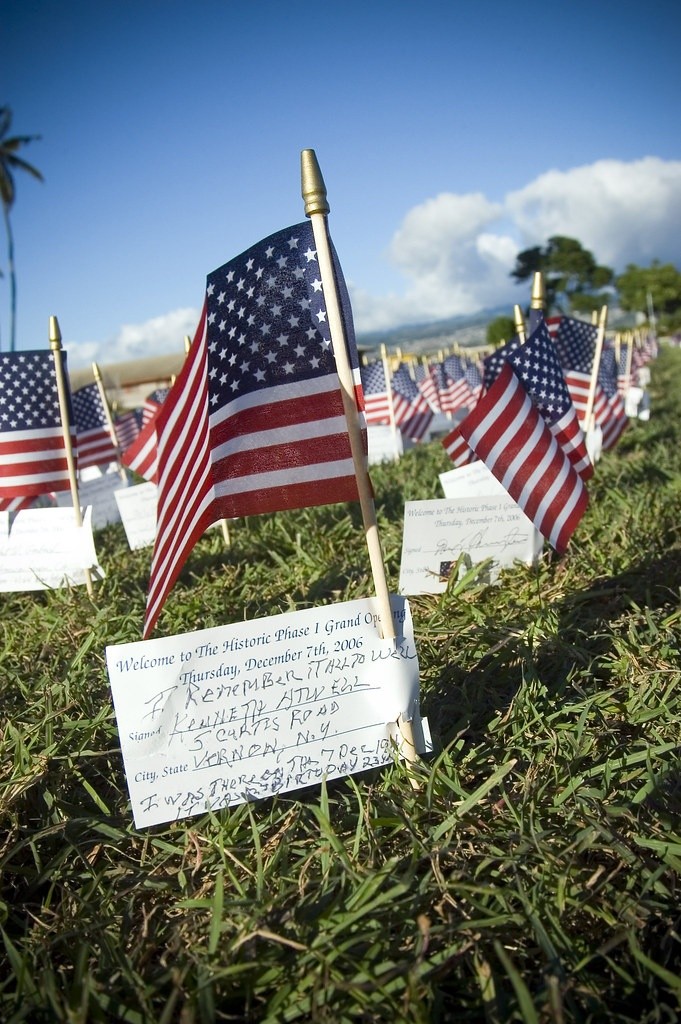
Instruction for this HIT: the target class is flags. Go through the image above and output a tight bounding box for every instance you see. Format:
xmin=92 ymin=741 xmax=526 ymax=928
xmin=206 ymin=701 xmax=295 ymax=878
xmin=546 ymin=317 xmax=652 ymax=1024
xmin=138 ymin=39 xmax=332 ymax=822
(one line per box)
xmin=362 ymin=309 xmax=658 ymax=557
xmin=120 ymin=219 xmax=368 ymax=640
xmin=0 ymin=349 xmax=177 ymax=511
